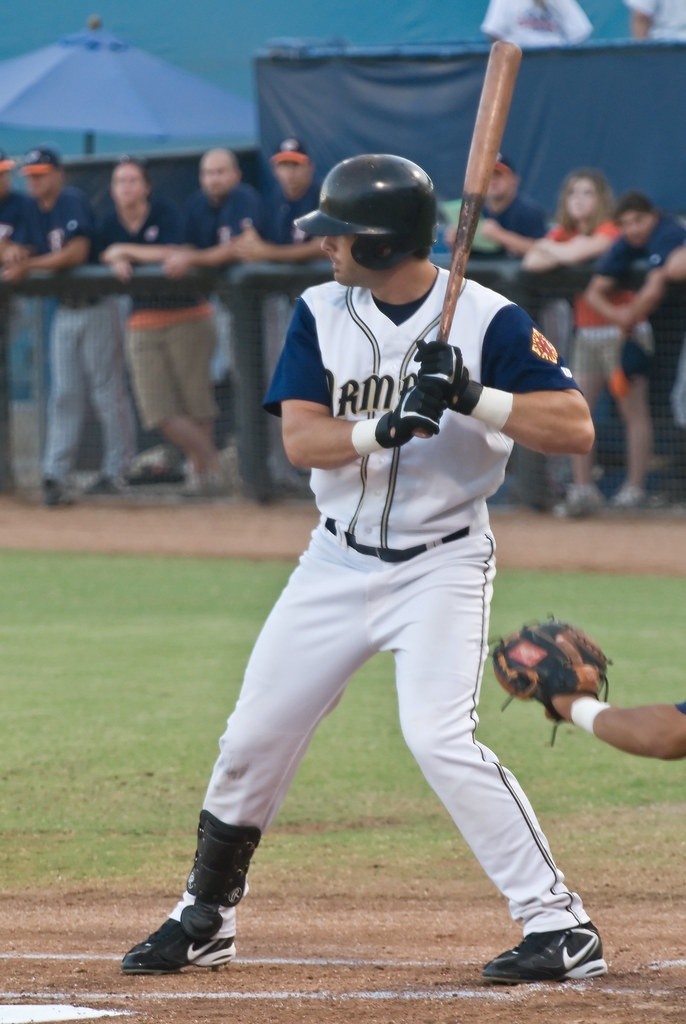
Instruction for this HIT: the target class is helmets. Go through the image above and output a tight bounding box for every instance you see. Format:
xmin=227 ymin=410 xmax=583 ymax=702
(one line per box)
xmin=292 ymin=154 xmax=438 ymax=270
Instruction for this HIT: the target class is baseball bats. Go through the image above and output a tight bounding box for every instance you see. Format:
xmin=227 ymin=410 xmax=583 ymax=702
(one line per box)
xmin=412 ymin=40 xmax=522 ymax=439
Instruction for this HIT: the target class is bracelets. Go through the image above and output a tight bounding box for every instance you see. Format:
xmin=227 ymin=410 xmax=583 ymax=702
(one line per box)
xmin=271 ymin=245 xmax=280 ymax=263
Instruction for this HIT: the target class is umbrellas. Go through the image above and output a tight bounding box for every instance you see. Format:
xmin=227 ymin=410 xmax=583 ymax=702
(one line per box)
xmin=0 ymin=13 xmax=260 ymax=158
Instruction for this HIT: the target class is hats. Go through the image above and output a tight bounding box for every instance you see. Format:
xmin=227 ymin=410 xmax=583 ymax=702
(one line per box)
xmin=272 ymin=137 xmax=309 ymax=163
xmin=19 ymin=148 xmax=59 ymax=177
xmin=0 ymin=149 xmax=17 ymax=172
xmin=491 ymin=153 xmax=513 ymax=177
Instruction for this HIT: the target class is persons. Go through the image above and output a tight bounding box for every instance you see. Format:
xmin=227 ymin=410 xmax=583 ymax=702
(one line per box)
xmin=0 ymin=148 xmax=137 ymax=501
xmin=478 ymin=0 xmax=595 ymax=50
xmin=492 ymin=616 xmax=686 ymax=760
xmin=120 ymin=156 xmax=610 ymax=986
xmin=621 ymin=0 xmax=686 ymax=42
xmin=87 ymin=157 xmax=231 ymax=499
xmin=229 ymin=135 xmax=332 ymax=500
xmin=162 ymin=149 xmax=298 ymax=497
xmin=445 ymin=148 xmax=573 ymax=504
xmin=518 ymin=163 xmax=652 ymax=521
xmin=587 ymin=193 xmax=686 ymax=504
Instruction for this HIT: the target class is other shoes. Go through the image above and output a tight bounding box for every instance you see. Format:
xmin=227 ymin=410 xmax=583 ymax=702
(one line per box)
xmin=42 ymin=482 xmax=71 ymax=511
xmin=550 ymin=483 xmax=605 ymax=518
xmin=608 ymin=484 xmax=650 ymax=510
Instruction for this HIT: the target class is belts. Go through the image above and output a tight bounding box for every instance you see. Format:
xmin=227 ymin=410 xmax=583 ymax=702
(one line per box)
xmin=323 ymin=519 xmax=471 ymax=563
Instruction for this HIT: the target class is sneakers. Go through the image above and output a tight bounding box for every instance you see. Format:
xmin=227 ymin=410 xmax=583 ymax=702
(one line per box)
xmin=122 ymin=917 xmax=237 ymax=974
xmin=481 ymin=921 xmax=607 ymax=983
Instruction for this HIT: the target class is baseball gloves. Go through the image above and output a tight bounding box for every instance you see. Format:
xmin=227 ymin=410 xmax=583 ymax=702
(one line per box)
xmin=492 ymin=620 xmax=609 ymax=704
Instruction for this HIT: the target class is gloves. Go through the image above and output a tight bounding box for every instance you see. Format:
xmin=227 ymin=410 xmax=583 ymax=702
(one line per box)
xmin=414 ymin=339 xmax=483 ymax=417
xmin=376 ymin=385 xmax=444 ymax=449
